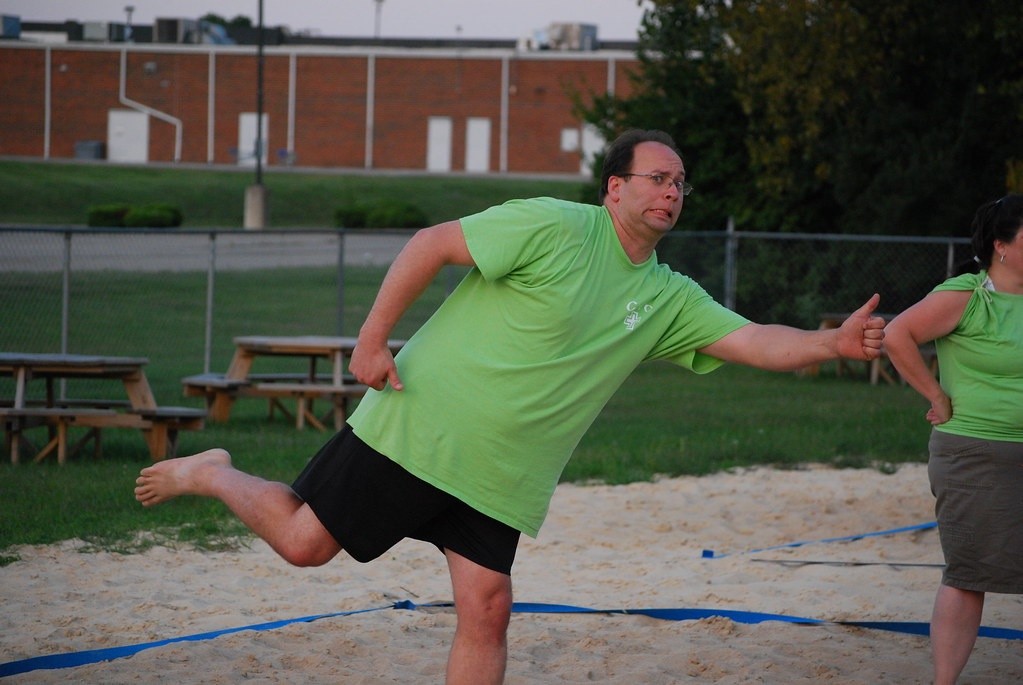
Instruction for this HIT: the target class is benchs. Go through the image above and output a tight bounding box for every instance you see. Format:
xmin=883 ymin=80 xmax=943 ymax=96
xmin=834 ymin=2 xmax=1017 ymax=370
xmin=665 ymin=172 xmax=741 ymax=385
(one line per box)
xmin=0 ymin=397 xmax=208 ymax=467
xmin=876 ymin=348 xmax=941 ymax=388
xmin=180 ymin=369 xmax=367 ymax=431
xmin=210 ymin=333 xmax=410 ymax=434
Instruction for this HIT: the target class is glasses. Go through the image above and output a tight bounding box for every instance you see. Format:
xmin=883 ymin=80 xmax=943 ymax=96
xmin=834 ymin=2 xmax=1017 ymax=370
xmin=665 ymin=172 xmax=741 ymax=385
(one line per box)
xmin=620 ymin=172 xmax=693 ymax=195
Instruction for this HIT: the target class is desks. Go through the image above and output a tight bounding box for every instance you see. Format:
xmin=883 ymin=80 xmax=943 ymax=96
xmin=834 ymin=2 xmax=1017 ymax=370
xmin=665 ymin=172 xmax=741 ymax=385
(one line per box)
xmin=0 ymin=352 xmax=175 ymax=468
xmin=810 ymin=312 xmax=901 ymax=386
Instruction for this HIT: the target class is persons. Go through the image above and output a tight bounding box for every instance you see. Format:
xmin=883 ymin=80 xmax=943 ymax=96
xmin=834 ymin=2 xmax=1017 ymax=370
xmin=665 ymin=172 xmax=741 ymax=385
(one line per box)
xmin=881 ymin=192 xmax=1023 ymax=685
xmin=133 ymin=130 xmax=885 ymax=685
